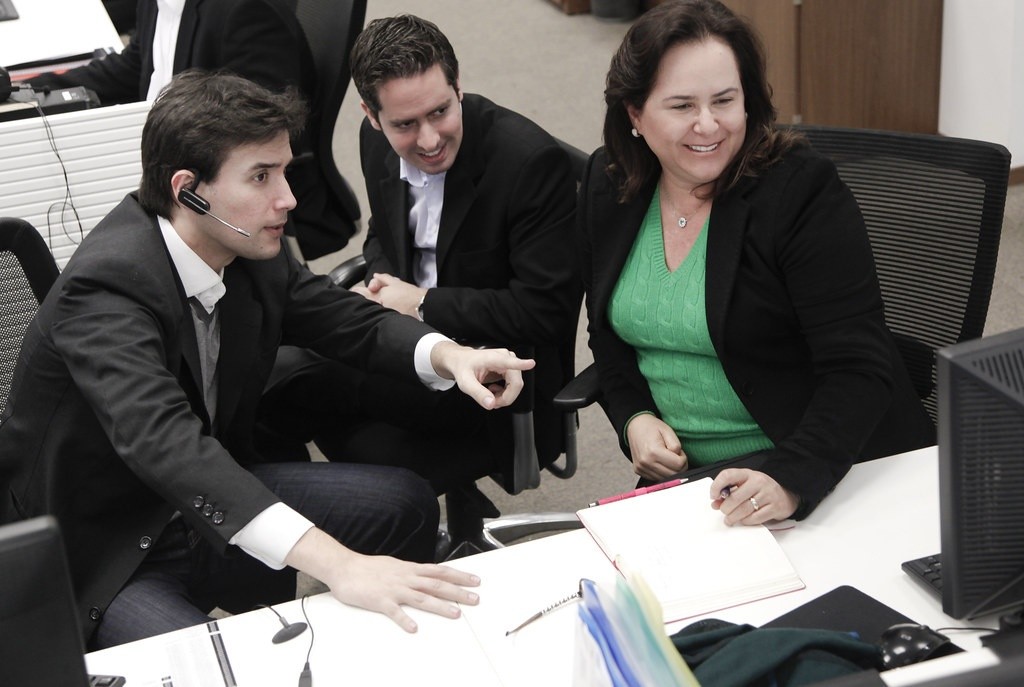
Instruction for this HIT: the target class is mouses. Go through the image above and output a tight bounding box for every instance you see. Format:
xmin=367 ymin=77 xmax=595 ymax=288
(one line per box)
xmin=878 ymin=623 xmax=950 ymax=671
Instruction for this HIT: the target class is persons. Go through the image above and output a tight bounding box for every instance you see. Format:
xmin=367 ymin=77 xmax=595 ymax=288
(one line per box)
xmin=582 ymin=0 xmax=937 ymax=528
xmin=0 ymin=0 xmax=355 ymax=274
xmin=0 ymin=69 xmax=539 ymax=650
xmin=271 ymin=10 xmax=581 ymax=496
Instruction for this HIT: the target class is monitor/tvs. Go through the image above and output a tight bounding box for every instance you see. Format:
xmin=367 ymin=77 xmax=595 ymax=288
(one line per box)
xmin=935 ymin=326 xmax=1024 ymax=635
xmin=0 ymin=514 xmax=93 ymax=687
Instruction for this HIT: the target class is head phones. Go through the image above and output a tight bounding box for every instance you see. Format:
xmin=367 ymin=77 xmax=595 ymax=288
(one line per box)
xmin=0 ymin=67 xmax=12 ymax=103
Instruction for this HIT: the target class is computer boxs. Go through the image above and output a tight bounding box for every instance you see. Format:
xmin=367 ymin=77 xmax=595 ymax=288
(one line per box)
xmin=0 ymin=86 xmax=100 ymax=122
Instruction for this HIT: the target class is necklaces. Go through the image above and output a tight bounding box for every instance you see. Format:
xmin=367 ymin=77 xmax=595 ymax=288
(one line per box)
xmin=660 ymin=172 xmax=713 ymax=228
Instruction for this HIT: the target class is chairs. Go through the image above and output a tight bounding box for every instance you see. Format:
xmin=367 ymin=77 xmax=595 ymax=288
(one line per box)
xmin=0 ymin=216 xmax=306 ymax=617
xmin=327 ymin=139 xmax=592 ymax=566
xmin=553 ymin=126 xmax=1012 ymax=483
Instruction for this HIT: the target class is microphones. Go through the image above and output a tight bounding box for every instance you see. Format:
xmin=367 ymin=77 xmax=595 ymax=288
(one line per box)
xmin=12 ymin=85 xmax=51 ymax=95
xmin=178 ymin=188 xmax=250 ymax=238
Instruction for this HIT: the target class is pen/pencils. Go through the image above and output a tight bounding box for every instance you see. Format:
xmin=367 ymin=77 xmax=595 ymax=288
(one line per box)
xmin=720 ymin=487 xmax=730 ymax=498
xmin=588 ymin=477 xmax=689 ymax=507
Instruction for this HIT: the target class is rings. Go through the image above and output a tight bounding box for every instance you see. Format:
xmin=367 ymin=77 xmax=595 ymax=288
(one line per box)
xmin=750 ymin=497 xmax=759 ymax=510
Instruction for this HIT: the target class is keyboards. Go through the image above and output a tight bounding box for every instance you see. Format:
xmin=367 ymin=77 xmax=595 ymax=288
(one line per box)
xmin=88 ymin=673 xmax=126 ymax=687
xmin=902 ymin=553 xmax=942 ymax=604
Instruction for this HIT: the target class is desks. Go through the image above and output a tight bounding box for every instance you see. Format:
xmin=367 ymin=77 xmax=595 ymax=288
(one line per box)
xmin=81 ymin=443 xmax=1011 ymax=687
